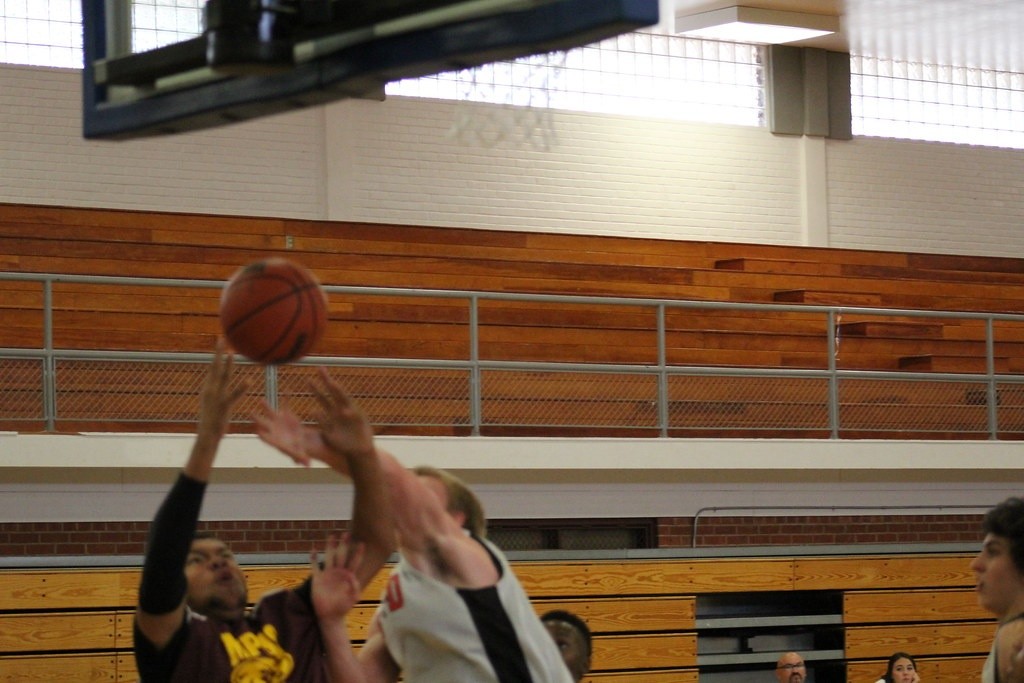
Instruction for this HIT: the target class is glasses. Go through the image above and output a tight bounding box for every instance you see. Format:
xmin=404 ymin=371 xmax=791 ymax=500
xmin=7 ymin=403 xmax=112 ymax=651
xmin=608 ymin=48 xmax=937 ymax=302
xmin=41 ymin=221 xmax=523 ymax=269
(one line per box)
xmin=778 ymin=662 xmax=804 ymax=668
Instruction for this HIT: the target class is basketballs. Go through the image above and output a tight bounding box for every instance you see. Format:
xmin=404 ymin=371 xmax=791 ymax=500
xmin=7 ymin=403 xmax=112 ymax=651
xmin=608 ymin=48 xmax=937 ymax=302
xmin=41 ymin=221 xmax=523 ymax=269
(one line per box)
xmin=219 ymin=257 xmax=327 ymax=366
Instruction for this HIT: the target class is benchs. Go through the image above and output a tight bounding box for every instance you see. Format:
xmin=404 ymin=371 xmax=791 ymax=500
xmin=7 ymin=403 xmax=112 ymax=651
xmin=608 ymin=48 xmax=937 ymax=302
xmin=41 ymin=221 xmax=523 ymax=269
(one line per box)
xmin=0 ymin=202 xmax=1024 ymax=439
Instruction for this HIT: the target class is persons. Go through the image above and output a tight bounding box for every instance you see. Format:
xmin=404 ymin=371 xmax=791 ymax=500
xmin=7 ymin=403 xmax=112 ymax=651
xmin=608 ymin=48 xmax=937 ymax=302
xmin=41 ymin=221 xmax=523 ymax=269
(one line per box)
xmin=875 ymin=652 xmax=920 ymax=683
xmin=130 ymin=337 xmax=394 ymax=683
xmin=774 ymin=653 xmax=807 ymax=683
xmin=969 ymin=496 xmax=1024 ymax=683
xmin=249 ymin=398 xmax=574 ymax=683
xmin=539 ymin=609 xmax=593 ymax=683
